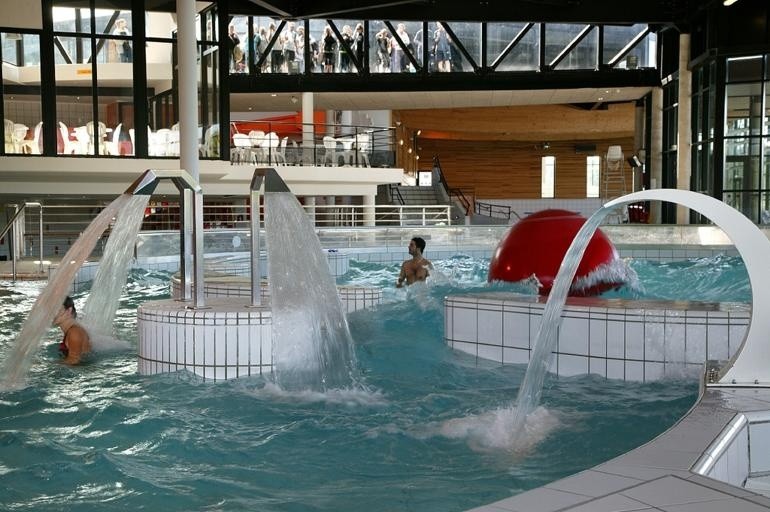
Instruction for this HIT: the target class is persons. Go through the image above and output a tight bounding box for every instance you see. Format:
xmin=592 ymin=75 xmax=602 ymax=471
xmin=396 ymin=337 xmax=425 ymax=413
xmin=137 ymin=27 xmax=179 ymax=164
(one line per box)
xmin=52 ymin=295 xmax=91 ymax=368
xmin=113 ymin=19 xmax=134 ymax=62
xmin=202 ymin=20 xmax=463 ymax=73
xmin=396 ymin=237 xmax=433 ymax=289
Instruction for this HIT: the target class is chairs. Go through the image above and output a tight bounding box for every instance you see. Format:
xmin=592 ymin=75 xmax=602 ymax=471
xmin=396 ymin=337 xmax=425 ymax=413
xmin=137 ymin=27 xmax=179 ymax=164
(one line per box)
xmin=4 ymin=118 xmax=221 ymax=159
xmin=228 ymin=128 xmax=374 ymax=169
